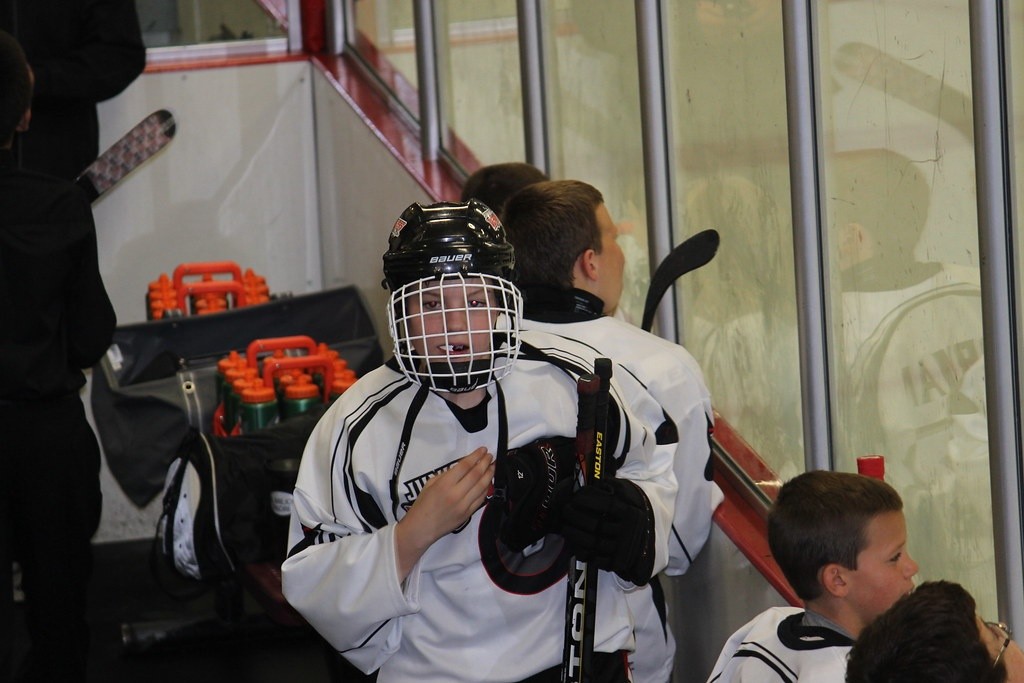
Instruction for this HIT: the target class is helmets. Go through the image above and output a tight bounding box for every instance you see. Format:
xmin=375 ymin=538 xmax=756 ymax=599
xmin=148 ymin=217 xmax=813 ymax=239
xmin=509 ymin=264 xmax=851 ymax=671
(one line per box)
xmin=382 ymin=198 xmax=523 ymax=392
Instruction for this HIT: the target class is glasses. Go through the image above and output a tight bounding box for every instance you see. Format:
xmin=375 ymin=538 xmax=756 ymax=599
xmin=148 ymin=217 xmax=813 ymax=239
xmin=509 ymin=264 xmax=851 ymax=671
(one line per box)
xmin=983 ymin=621 xmax=1012 ymax=673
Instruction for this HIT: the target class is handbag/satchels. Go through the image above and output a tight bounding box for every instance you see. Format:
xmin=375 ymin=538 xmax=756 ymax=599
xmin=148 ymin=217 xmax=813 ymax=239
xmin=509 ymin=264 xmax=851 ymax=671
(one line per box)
xmin=151 ymin=409 xmax=328 ymax=588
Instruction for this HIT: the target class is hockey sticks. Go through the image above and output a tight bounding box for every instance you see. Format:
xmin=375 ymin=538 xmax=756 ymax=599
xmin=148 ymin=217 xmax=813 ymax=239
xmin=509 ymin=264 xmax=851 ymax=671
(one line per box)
xmin=557 ymin=355 xmax=615 ymax=683
xmin=639 ymin=225 xmax=722 ymax=332
xmin=72 ymin=106 xmax=181 ymax=206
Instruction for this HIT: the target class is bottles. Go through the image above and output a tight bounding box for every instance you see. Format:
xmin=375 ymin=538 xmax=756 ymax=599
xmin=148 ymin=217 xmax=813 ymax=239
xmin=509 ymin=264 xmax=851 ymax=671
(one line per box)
xmin=145 ymin=267 xmax=270 ymax=319
xmin=215 ymin=336 xmax=360 ymax=436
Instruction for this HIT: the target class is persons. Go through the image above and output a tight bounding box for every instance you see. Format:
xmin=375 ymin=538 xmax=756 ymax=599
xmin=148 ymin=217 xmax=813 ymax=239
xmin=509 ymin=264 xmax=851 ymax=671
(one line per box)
xmin=615 ymin=148 xmax=997 ymax=628
xmin=280 ymin=162 xmax=726 ymax=683
xmin=0 ymin=0 xmax=146 ymax=683
xmin=846 ymin=580 xmax=1024 ymax=683
xmin=707 ymin=470 xmax=918 ymax=683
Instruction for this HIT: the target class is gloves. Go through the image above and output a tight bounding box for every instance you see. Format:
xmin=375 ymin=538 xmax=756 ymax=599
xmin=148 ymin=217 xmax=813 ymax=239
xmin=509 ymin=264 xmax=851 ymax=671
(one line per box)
xmin=548 ymin=477 xmax=655 ymax=586
